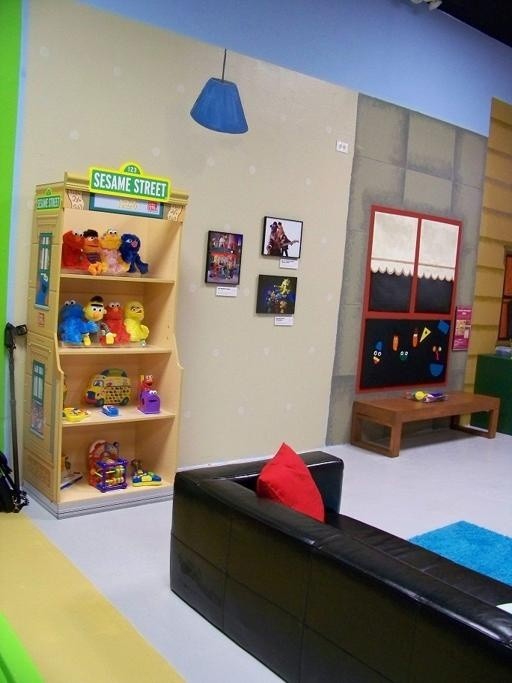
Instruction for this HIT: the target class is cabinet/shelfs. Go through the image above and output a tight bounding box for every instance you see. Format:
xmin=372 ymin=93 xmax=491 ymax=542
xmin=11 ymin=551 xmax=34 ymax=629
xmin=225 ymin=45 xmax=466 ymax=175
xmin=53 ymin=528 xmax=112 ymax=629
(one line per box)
xmin=20 ymin=162 xmax=190 ymax=519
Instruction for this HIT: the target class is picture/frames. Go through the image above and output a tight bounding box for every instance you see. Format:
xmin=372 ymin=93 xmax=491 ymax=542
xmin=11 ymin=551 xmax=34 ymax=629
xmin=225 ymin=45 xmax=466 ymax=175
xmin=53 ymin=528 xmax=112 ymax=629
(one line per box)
xmin=204 ymin=215 xmax=303 ymax=315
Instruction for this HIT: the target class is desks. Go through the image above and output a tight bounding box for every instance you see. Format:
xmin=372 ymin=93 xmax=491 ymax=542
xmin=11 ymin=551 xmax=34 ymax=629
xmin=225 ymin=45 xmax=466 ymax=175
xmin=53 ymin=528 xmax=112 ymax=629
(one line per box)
xmin=350 ymin=390 xmax=500 ymax=457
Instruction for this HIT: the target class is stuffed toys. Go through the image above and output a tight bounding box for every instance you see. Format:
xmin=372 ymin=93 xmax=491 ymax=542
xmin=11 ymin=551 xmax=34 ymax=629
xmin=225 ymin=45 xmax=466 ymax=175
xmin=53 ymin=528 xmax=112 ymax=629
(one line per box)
xmin=122 ymin=300 xmax=150 ymax=341
xmin=80 ymin=229 xmax=104 ymax=273
xmin=98 ymin=228 xmax=131 ymax=274
xmin=58 ymin=298 xmax=98 ymax=343
xmin=119 ymin=233 xmax=149 ymax=274
xmin=99 ymin=301 xmax=131 ymax=344
xmin=59 ymin=229 xmax=85 ymax=269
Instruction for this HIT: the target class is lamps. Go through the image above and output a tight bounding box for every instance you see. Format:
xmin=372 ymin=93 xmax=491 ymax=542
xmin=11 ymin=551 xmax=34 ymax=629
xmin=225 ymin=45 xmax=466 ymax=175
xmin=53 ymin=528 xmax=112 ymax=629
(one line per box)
xmin=189 ymin=49 xmax=250 ymax=135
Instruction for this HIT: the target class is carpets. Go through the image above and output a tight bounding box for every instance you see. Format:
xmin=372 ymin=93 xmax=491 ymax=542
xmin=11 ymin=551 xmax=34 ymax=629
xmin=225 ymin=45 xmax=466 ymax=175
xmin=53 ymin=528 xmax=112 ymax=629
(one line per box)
xmin=406 ymin=519 xmax=512 ymax=587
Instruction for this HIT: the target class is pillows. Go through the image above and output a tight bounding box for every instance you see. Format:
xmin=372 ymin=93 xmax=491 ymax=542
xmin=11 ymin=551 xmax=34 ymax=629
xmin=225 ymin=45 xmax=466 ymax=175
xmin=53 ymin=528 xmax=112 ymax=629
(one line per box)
xmin=256 ymin=442 xmax=325 ymax=526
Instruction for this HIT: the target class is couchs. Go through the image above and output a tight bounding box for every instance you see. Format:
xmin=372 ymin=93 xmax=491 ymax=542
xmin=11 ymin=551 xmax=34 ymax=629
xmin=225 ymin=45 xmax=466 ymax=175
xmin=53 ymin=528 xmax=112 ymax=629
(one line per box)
xmin=170 ymin=449 xmax=512 ymax=682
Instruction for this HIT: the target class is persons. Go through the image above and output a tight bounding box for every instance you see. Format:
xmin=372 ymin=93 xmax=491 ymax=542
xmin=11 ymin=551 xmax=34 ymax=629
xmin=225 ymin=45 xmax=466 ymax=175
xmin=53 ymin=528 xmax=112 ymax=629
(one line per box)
xmin=82 ymin=293 xmax=117 ymax=346
